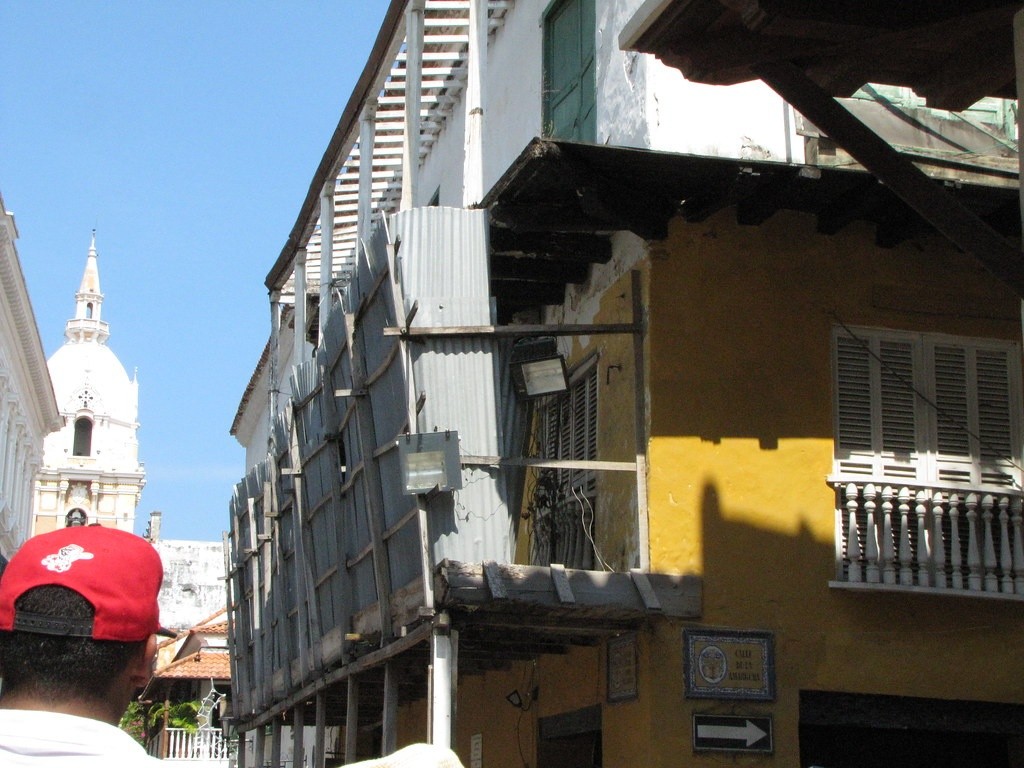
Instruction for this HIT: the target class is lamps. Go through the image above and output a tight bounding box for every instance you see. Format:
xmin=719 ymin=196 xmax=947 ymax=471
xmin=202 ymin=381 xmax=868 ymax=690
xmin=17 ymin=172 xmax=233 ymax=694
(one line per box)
xmin=397 ymin=428 xmax=462 ymax=494
xmin=509 ymin=355 xmax=571 ymax=401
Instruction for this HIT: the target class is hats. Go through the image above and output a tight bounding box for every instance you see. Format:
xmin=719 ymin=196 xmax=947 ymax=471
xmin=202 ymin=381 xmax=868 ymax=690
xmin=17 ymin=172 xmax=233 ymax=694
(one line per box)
xmin=0 ymin=525 xmax=178 ymax=643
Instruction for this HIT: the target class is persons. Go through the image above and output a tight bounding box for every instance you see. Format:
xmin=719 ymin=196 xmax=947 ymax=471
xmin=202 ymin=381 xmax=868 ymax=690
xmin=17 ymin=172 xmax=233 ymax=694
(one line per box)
xmin=0 ymin=526 xmax=182 ymax=768
